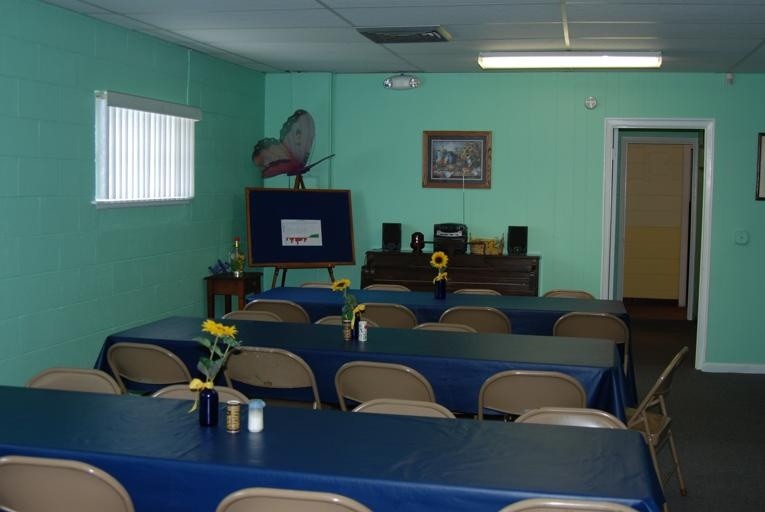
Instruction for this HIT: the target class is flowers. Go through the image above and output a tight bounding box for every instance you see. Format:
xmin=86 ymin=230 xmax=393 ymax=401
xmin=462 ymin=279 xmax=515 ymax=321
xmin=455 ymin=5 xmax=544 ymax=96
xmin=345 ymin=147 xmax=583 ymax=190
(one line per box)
xmin=431 ymin=251 xmax=450 ymax=283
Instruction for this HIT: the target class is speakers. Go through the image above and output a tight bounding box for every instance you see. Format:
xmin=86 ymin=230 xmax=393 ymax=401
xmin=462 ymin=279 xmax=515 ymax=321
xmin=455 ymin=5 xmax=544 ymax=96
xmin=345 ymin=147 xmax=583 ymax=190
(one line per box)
xmin=508 ymin=226 xmax=527 ymax=256
xmin=433 ymin=223 xmax=467 ymax=253
xmin=382 ymin=223 xmax=402 ymax=251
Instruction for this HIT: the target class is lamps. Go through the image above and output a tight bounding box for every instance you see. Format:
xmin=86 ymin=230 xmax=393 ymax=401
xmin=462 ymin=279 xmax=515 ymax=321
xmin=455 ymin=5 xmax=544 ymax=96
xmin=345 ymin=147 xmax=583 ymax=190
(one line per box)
xmin=382 ymin=73 xmax=421 ymax=90
xmin=477 ymin=50 xmax=664 ymax=70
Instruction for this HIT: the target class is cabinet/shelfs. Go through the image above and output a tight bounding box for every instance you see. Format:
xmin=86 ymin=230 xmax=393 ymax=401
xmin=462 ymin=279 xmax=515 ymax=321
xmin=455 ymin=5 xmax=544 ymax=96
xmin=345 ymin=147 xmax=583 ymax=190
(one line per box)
xmin=360 ymin=250 xmax=541 ymax=297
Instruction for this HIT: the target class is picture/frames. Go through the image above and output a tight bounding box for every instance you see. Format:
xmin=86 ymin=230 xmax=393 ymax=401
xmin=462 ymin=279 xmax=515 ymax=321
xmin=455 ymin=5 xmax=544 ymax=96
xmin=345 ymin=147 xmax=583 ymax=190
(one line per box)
xmin=421 ymin=130 xmax=492 ymax=189
xmin=754 ymin=132 xmax=765 ymax=201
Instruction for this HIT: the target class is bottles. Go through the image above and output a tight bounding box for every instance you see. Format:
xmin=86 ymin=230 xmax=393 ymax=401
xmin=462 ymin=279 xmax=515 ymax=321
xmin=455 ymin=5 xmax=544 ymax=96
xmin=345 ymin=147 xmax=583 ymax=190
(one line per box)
xmin=359 ymin=320 xmax=368 ymax=343
xmin=247 ymin=398 xmax=266 ymax=434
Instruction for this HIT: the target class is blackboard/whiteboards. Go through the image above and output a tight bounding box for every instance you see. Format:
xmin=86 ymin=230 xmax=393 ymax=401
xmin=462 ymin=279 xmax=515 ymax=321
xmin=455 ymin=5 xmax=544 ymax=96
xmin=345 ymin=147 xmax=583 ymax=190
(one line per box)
xmin=245 ymin=186 xmax=356 ymax=267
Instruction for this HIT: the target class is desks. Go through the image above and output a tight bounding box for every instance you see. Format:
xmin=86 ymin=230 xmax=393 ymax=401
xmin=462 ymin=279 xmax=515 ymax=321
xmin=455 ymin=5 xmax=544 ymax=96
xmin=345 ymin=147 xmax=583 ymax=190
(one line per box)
xmin=205 ymin=271 xmax=264 ymax=319
